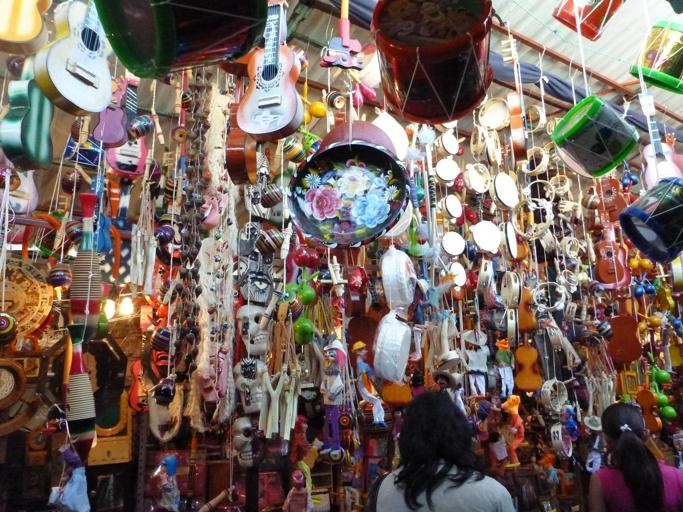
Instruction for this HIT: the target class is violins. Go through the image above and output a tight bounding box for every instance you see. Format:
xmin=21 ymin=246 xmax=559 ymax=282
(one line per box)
xmin=515 ymin=235 xmax=542 ymax=390
xmin=637 ymin=356 xmax=662 ymax=433
xmin=592 ymin=177 xmax=642 ymax=364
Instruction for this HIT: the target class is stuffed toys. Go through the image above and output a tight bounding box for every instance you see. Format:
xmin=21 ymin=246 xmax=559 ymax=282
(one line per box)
xmin=288 ymin=415 xmax=313 ymax=466
xmin=559 ymin=405 xmax=580 ymax=438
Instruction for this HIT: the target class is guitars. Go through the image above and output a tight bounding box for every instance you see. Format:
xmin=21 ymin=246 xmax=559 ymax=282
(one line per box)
xmin=654 ymin=261 xmax=675 ymax=312
xmin=0 ymin=0 xmax=148 ymax=214
xmin=226 ymin=0 xmax=304 ymax=184
xmin=129 ymin=331 xmax=153 ymax=412
xmin=639 ymin=96 xmax=683 ymax=190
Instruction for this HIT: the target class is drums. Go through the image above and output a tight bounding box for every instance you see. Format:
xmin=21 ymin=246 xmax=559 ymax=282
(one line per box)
xmin=370 ymin=0 xmax=495 ymax=124
xmin=630 ymin=19 xmax=683 ymax=93
xmin=434 ymin=98 xmax=520 ymax=352
xmin=552 ymin=0 xmax=628 ymax=41
xmin=94 ymin=0 xmax=267 ymax=78
xmin=619 ymin=176 xmax=683 ymax=262
xmin=552 ymin=95 xmax=639 ymax=177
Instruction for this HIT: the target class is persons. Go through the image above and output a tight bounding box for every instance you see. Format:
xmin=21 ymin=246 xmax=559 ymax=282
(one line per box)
xmin=536 ymin=453 xmax=565 ymax=500
xmin=528 ymin=405 xmax=551 ymax=454
xmin=498 ymin=395 xmax=526 ymax=469
xmin=473 ymin=398 xmax=502 ymax=444
xmin=279 ymin=469 xmax=312 ymax=511
xmin=374 ymin=388 xmax=515 ymax=511
xmin=583 ymin=400 xmax=683 ymax=511
xmin=494 ymin=337 xmax=518 ymax=396
xmin=317 ymin=339 xmax=348 ymax=464
xmin=350 ymin=338 xmax=388 ymax=431
xmin=147 ymin=454 xmax=181 ymax=511
